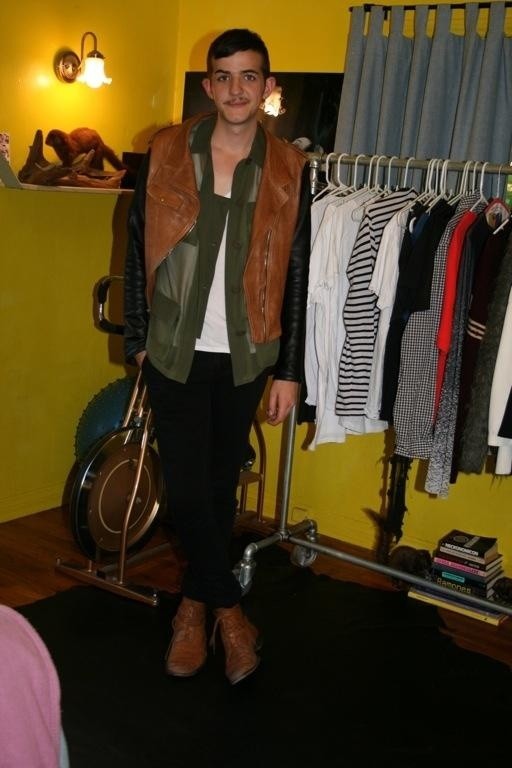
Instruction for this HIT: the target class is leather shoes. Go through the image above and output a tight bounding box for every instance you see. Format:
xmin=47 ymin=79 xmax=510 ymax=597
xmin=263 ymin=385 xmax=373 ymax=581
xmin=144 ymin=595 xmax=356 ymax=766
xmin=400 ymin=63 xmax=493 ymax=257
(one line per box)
xmin=165 ymin=597 xmax=262 ymax=685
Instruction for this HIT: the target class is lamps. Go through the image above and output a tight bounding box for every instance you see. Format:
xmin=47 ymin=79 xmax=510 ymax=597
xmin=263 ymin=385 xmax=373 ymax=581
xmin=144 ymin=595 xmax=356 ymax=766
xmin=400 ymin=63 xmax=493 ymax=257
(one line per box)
xmin=57 ymin=33 xmax=114 ymax=88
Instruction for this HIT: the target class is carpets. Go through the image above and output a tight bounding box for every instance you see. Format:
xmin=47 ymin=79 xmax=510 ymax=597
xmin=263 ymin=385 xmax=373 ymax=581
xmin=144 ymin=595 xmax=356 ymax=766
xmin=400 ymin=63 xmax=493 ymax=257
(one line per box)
xmin=12 ymin=525 xmax=511 ymax=767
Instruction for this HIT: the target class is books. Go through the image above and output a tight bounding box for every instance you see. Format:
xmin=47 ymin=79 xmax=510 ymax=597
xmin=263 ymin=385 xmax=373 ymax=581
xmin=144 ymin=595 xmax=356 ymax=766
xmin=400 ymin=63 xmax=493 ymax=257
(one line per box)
xmin=406 ymin=530 xmax=512 ymax=628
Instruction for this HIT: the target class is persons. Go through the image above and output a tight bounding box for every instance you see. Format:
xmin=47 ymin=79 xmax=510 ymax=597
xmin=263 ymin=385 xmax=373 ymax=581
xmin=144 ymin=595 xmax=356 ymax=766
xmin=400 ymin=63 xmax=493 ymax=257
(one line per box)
xmin=122 ymin=28 xmax=311 ymax=689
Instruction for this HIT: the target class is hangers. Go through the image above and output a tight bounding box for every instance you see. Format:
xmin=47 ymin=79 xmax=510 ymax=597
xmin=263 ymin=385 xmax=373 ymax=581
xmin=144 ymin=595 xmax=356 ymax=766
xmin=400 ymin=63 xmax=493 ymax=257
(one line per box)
xmin=310 ymin=151 xmax=511 ymax=238
